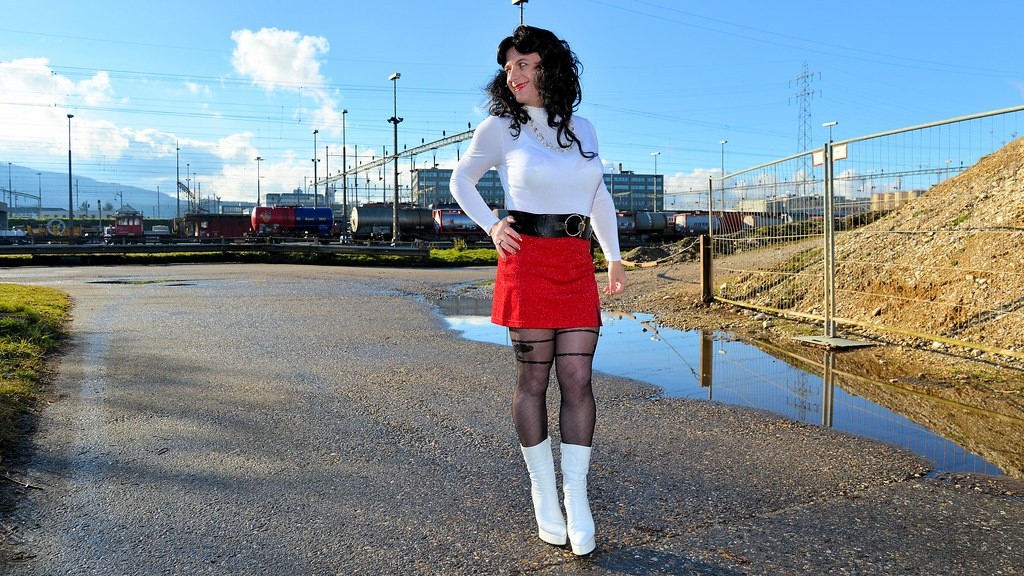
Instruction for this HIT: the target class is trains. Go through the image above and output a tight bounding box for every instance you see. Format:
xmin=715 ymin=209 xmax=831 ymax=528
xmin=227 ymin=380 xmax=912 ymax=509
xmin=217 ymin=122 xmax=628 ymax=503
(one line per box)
xmin=8 ymin=204 xmax=855 ymax=245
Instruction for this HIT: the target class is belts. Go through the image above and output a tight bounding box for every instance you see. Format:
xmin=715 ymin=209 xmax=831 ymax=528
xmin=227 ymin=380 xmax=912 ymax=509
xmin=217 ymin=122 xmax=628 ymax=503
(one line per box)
xmin=507 ymin=209 xmax=591 ymax=239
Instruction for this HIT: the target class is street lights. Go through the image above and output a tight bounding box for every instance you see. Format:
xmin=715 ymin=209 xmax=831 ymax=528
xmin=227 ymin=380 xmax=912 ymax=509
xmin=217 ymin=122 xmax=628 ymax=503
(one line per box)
xmin=341 ymin=109 xmax=349 ymax=231
xmin=512 ymin=0 xmax=530 ymax=25
xmin=311 ymin=129 xmax=321 ymax=208
xmin=387 ymin=72 xmax=404 ymax=242
xmin=176 ymin=147 xmax=180 ymax=218
xmin=198 ymin=182 xmax=200 ymax=205
xmin=185 ymin=164 xmax=191 ymax=213
xmin=67 ymin=113 xmax=74 ymax=238
xmin=193 ymin=173 xmax=196 ymax=203
xmin=254 ymin=157 xmax=265 ymax=206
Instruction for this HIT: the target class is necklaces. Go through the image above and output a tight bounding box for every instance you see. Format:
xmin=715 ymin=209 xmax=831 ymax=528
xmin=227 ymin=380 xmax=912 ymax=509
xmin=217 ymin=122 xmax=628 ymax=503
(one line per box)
xmin=523 ymin=105 xmax=574 ymax=151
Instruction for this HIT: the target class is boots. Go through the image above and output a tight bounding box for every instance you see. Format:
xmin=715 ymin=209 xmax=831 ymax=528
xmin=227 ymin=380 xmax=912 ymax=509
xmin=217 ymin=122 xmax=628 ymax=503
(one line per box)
xmin=559 ymin=442 xmax=596 ymax=556
xmin=520 ymin=436 xmax=568 ymax=546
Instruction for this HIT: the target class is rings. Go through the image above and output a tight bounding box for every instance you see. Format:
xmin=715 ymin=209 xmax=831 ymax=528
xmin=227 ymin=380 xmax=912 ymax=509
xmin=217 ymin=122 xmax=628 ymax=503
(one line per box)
xmin=498 ymin=240 xmax=503 ymax=245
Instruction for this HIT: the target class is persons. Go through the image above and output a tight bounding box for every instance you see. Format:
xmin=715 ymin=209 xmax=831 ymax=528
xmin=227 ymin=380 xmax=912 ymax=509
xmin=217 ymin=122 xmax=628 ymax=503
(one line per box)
xmin=450 ymin=27 xmax=626 ymax=556
